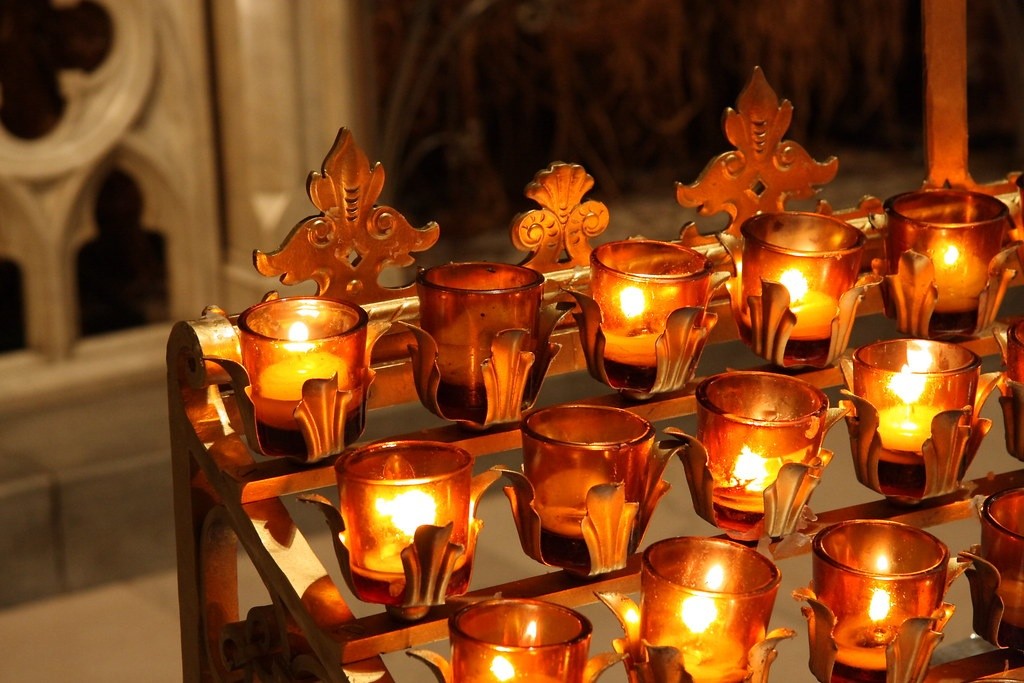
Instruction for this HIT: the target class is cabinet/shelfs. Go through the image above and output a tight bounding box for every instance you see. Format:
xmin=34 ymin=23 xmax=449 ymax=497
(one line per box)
xmin=167 ymin=66 xmax=1024 ymax=683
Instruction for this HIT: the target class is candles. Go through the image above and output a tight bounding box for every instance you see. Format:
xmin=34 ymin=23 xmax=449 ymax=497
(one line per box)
xmin=258 ymin=321 xmax=349 ymax=400
xmin=833 ymin=589 xmax=911 ymax=670
xmin=363 ymin=489 xmax=467 ymax=574
xmin=779 ymin=268 xmax=836 ymax=339
xmin=928 ymin=240 xmax=987 ymax=311
xmin=656 ymin=596 xmax=746 ymax=683
xmin=603 ymin=287 xmax=665 ymax=366
xmin=876 ymin=363 xmax=948 ymax=452
